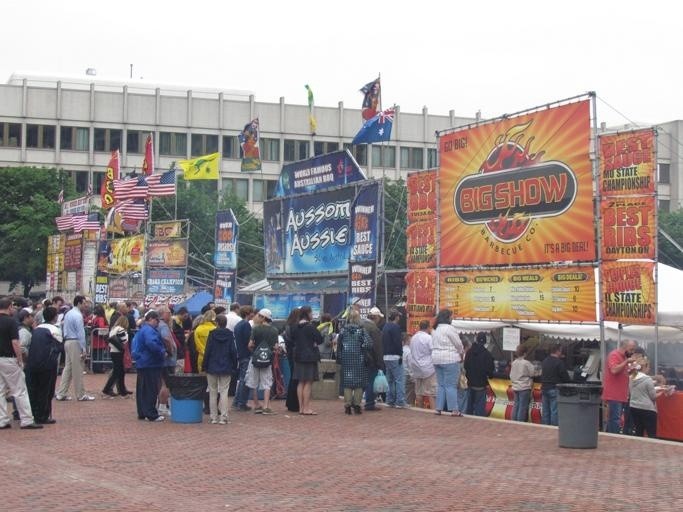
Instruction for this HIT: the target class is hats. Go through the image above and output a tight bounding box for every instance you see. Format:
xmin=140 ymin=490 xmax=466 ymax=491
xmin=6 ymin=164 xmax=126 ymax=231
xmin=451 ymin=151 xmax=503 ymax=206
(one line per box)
xmin=369 ymin=307 xmax=403 ymax=319
xmin=258 ymin=309 xmax=271 ymax=319
xmin=18 ymin=307 xmax=36 ymax=319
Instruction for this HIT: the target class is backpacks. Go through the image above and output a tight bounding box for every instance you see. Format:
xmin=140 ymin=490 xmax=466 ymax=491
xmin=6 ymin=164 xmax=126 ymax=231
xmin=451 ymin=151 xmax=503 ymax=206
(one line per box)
xmin=252 ymin=339 xmax=273 ymax=367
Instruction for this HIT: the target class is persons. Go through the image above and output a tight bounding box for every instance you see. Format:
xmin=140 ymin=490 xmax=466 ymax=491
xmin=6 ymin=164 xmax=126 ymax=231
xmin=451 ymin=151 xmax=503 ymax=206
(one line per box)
xmin=56 ymin=295 xmax=96 ymax=401
xmin=12 ymin=311 xmax=33 ymax=421
xmin=26 ymin=307 xmax=63 ymax=426
xmin=622 ymin=346 xmax=664 ymax=435
xmin=337 ymin=311 xmax=374 ymax=416
xmin=626 ymin=354 xmax=676 ymax=439
xmin=431 ymin=308 xmax=464 ymax=416
xmin=359 ymin=307 xmax=382 ymax=411
xmin=508 ymin=345 xmax=541 ymax=422
xmin=201 ymin=314 xmax=237 ymax=424
xmin=101 ymin=317 xmax=135 ymax=397
xmin=600 ymin=338 xmax=638 ymax=434
xmin=380 ymin=311 xmax=470 ymax=415
xmin=131 ymin=300 xmax=337 ymax=414
xmin=462 ymin=330 xmax=493 ymax=417
xmin=1 ymin=289 xmax=142 ymax=374
xmin=540 ymin=342 xmax=569 ymax=426
xmin=0 ymin=298 xmax=42 ymax=431
xmin=131 ymin=311 xmax=168 ymax=422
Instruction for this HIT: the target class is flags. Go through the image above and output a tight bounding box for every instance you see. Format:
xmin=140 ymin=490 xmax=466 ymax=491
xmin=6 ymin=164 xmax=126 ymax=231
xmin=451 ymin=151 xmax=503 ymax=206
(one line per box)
xmin=54 ymin=71 xmax=396 ymax=238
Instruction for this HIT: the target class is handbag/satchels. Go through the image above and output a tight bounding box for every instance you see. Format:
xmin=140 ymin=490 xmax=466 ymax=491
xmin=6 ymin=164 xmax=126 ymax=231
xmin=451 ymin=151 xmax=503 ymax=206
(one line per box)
xmin=459 ymin=368 xmax=467 ymax=389
xmin=373 ymin=369 xmax=390 ymax=393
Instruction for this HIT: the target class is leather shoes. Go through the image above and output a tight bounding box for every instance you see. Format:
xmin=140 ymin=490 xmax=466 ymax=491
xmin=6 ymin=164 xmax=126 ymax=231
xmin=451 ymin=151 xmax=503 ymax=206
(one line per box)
xmin=20 ymin=418 xmax=55 ymax=428
xmin=346 ymin=405 xmax=361 ymax=414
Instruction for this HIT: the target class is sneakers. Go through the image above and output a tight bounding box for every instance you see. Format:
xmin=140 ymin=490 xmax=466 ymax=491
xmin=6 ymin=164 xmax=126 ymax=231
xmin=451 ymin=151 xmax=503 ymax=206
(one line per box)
xmin=77 ymin=395 xmax=95 ymax=400
xmin=56 ymin=395 xmax=72 ymax=400
xmin=145 ymin=415 xmax=164 ymax=421
xmin=207 ymin=418 xmax=231 ymax=424
xmin=232 ymin=402 xmax=276 ymax=414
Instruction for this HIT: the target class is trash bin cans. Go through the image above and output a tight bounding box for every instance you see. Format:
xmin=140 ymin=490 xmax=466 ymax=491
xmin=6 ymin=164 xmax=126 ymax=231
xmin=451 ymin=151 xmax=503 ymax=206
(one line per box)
xmin=555 ymin=383 xmax=604 ymax=449
xmin=166 ymin=374 xmax=208 ymax=423
